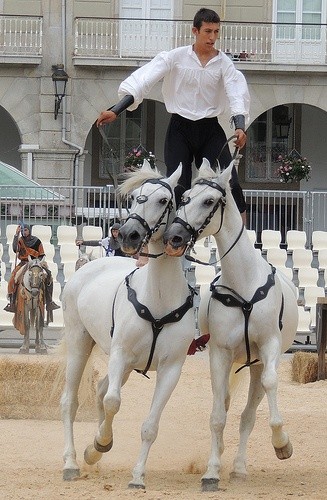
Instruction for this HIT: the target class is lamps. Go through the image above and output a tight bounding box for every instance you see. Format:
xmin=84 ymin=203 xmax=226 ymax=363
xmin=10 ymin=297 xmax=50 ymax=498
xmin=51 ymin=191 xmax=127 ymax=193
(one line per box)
xmin=275 ymin=104 xmax=292 ymax=139
xmin=52 ymin=64 xmax=68 ymax=120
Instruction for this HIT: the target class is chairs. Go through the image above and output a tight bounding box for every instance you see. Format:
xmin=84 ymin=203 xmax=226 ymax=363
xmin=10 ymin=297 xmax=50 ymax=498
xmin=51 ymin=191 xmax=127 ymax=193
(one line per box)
xmin=0 ymin=224 xmax=327 ymax=336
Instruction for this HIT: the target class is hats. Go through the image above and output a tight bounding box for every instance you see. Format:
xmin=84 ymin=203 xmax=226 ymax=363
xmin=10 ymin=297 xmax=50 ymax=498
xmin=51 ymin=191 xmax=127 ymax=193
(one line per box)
xmin=20 ymin=224 xmax=31 ymax=237
xmin=111 ymin=222 xmax=122 ymax=239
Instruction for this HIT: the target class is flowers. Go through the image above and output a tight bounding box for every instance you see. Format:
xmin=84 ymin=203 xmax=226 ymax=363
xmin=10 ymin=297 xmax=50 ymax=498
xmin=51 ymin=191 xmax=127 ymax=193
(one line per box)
xmin=277 ymin=154 xmax=311 ymax=184
xmin=124 ymin=148 xmax=155 ymax=173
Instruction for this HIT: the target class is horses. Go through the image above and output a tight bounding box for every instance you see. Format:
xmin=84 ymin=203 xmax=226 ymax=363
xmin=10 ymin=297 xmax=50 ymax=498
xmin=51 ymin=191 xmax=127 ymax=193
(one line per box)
xmin=7 ymin=252 xmax=51 ymax=356
xmin=161 ymin=154 xmax=299 ymax=492
xmin=60 ymin=158 xmax=196 ymax=490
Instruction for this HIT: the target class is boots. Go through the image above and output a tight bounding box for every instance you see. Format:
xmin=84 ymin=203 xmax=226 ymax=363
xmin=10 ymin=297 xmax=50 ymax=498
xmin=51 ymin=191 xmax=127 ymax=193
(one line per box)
xmin=6 ymin=293 xmax=16 ymax=311
xmin=45 ymin=281 xmax=60 ymax=311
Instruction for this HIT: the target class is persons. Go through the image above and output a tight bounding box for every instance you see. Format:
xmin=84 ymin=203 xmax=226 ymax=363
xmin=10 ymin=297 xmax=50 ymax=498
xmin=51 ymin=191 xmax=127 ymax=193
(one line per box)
xmin=5 ymin=224 xmax=60 ymax=312
xmin=97 ymin=9 xmax=250 ymax=227
xmin=76 ymin=223 xmax=124 ymax=257
